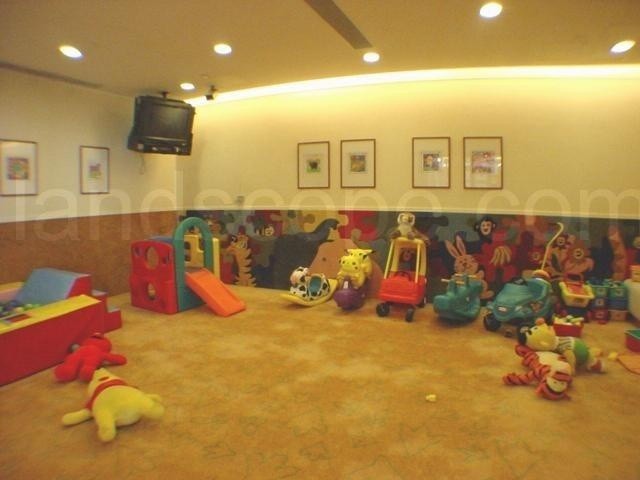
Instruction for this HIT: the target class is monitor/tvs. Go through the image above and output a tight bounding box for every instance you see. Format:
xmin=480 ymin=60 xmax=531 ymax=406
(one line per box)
xmin=134 ymin=99 xmax=192 ymax=147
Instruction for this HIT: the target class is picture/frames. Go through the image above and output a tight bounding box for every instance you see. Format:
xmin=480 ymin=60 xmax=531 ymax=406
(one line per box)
xmin=463 ymin=136 xmax=503 ymax=189
xmin=0 ymin=139 xmax=39 ymax=196
xmin=297 ymin=141 xmax=330 ymax=189
xmin=412 ymin=137 xmax=450 ymax=189
xmin=340 ymin=138 xmax=376 ymax=189
xmin=79 ymin=146 xmax=110 ymax=195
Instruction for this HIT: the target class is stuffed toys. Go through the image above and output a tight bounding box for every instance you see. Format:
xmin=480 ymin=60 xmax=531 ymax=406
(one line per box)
xmin=501 ymin=322 xmax=608 ymax=401
xmin=53 ymin=332 xmax=164 ymax=442
